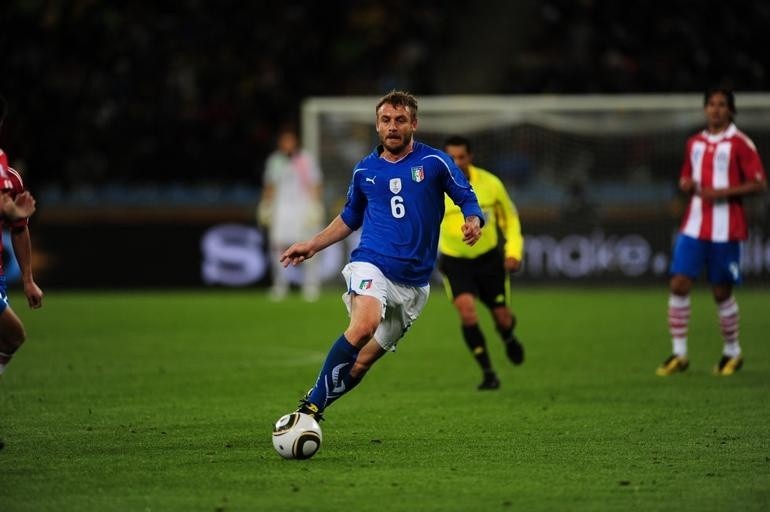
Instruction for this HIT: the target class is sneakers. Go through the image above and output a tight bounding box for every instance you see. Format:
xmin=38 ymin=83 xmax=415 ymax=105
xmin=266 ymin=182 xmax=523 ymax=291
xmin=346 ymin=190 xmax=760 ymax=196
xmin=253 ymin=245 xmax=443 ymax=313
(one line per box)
xmin=654 ymin=355 xmax=689 ymax=377
xmin=713 ymin=353 xmax=744 ymax=376
xmin=294 ymin=388 xmax=325 ymax=425
xmin=504 ymin=338 xmax=525 ymax=366
xmin=478 ymin=370 xmax=501 ymax=390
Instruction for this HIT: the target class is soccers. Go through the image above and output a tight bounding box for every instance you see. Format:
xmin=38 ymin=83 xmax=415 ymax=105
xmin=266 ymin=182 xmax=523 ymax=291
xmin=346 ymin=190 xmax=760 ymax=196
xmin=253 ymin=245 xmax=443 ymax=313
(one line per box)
xmin=272 ymin=413 xmax=322 ymax=458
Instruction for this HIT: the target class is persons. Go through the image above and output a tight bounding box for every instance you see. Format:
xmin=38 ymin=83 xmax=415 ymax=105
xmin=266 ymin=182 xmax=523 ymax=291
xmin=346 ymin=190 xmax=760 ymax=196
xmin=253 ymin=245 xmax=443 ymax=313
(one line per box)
xmin=279 ymin=89 xmax=485 ymax=423
xmin=656 ymin=86 xmax=769 ymax=378
xmin=0 ymin=191 xmax=38 ymax=219
xmin=437 ymin=134 xmax=525 ymax=391
xmin=257 ymin=128 xmax=325 ymax=302
xmin=1 ymin=149 xmax=44 ymax=449
